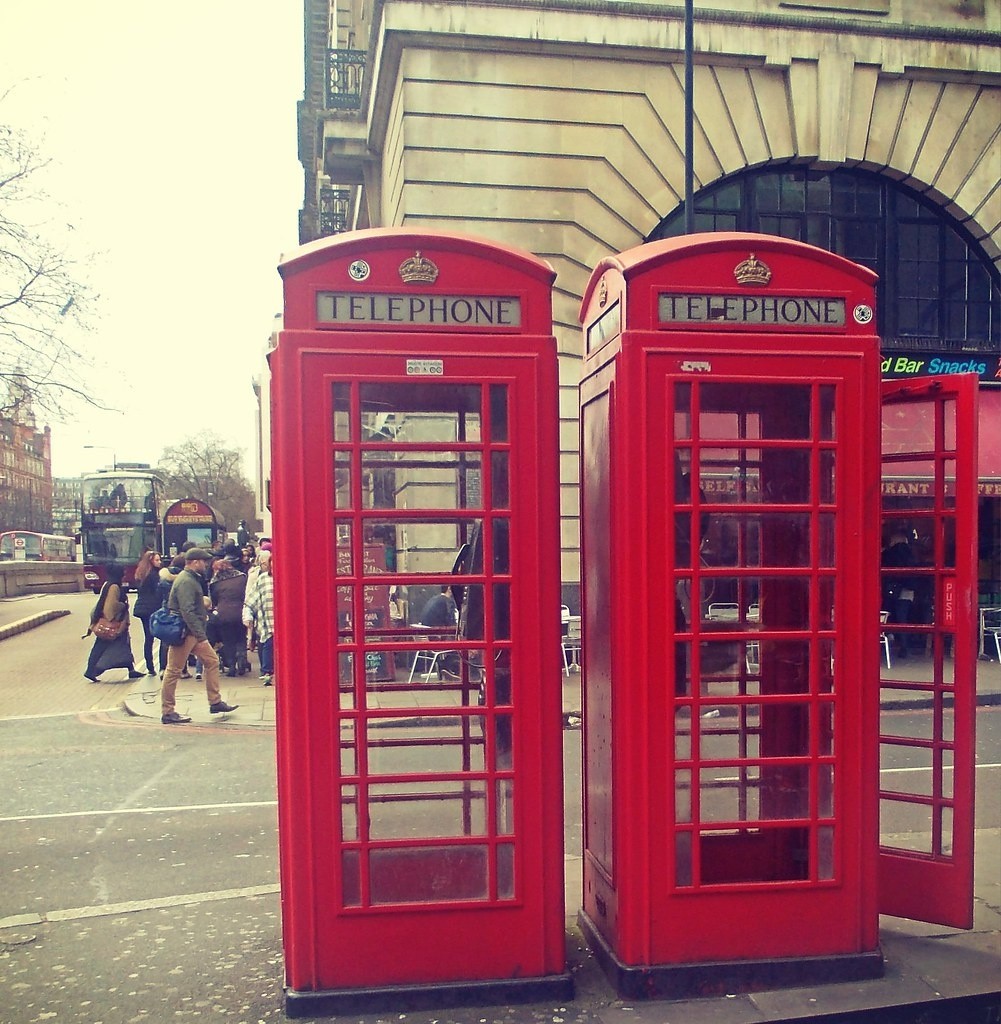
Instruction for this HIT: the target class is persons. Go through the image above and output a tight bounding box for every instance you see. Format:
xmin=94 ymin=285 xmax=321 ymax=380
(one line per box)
xmin=160 ymin=547 xmax=240 ymax=724
xmin=879 ymin=531 xmax=925 ymax=659
xmin=132 ymin=551 xmax=167 ymax=679
xmin=420 ymin=585 xmax=470 ymax=679
xmin=157 ymin=537 xmax=280 ymax=686
xmin=360 ymin=524 xmax=404 ymax=628
xmin=90 ymin=480 xmax=156 ymax=564
xmin=84 ymin=566 xmax=147 ymax=682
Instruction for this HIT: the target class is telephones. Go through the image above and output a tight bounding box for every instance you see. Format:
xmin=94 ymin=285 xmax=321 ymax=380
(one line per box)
xmin=673 ymin=450 xmax=710 ymax=567
xmin=450 ymin=517 xmax=508 ymax=640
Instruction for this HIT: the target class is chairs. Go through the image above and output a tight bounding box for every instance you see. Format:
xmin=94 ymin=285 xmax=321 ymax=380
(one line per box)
xmin=408 ymin=623 xmax=462 ymax=685
xmin=880 ymin=610 xmax=891 ymax=670
xmin=709 ymin=603 xmax=738 ymax=616
xmin=748 ymin=604 xmax=759 ymax=614
xmin=978 ymin=606 xmax=1001 ymax=664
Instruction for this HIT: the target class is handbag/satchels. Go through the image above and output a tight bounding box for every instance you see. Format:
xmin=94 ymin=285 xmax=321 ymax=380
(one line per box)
xmin=147 ymin=607 xmax=188 ymax=647
xmin=92 ymin=618 xmax=122 ymax=640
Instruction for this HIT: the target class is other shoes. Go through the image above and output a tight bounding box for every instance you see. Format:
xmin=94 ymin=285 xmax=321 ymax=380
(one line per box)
xmin=147 ymin=670 xmax=157 ymax=675
xmin=440 ymin=670 xmax=460 ymax=681
xmin=195 ymin=673 xmax=201 ymax=679
xmin=262 ymin=676 xmax=272 ymax=685
xmin=237 ymin=665 xmax=244 ymax=675
xmin=83 ymin=671 xmax=100 ymax=682
xmin=258 ymin=675 xmax=267 ymax=679
xmin=180 ymin=672 xmax=192 ymax=679
xmin=128 ymin=671 xmax=145 ymax=678
xmin=159 ymin=670 xmax=164 ymax=680
xmin=209 ymin=701 xmax=238 ymax=714
xmin=161 ymin=712 xmax=190 ymax=724
xmin=225 ymin=670 xmax=235 ymax=677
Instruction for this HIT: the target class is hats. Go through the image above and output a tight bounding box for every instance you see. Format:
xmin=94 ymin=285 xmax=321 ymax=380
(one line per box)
xmin=238 ymin=519 xmax=246 ymax=525
xmin=261 ymin=543 xmax=272 ymax=554
xmin=184 ymin=548 xmax=212 ymax=560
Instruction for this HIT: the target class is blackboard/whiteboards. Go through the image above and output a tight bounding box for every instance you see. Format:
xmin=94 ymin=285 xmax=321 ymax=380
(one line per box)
xmin=336 ymin=543 xmax=398 ymax=682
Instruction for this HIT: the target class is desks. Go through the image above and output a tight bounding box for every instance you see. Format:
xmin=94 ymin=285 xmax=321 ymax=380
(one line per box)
xmin=564 ymin=638 xmax=581 ymax=673
xmin=720 ymin=615 xmax=759 ymax=622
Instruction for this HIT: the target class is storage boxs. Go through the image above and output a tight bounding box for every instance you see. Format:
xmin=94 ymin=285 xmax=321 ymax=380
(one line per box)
xmin=567 ymin=629 xmax=581 ymax=638
xmin=568 ymin=621 xmax=581 ymax=630
